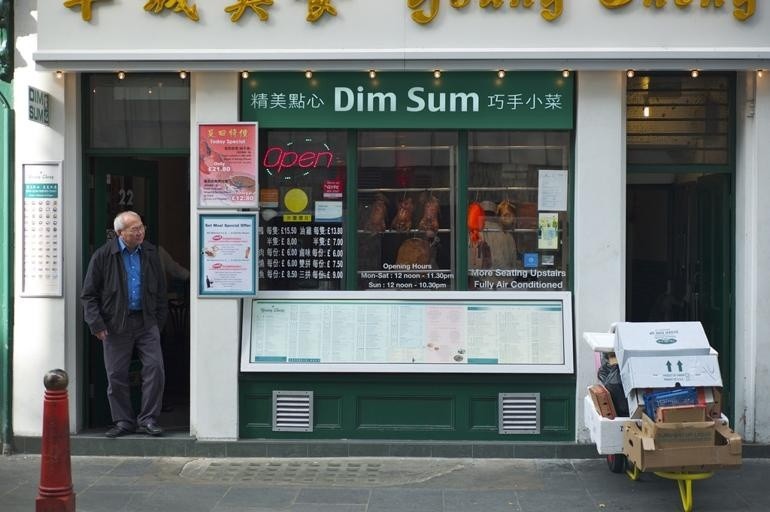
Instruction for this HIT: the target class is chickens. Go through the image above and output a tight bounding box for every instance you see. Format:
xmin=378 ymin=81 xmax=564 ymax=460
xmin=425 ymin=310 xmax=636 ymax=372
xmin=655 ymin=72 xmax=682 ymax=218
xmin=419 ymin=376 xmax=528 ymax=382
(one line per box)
xmin=390 ymin=197 xmax=413 ymax=233
xmin=496 ymin=198 xmax=516 ymax=230
xmin=365 ymin=194 xmax=386 ymax=237
xmin=420 ymin=196 xmax=441 ymax=233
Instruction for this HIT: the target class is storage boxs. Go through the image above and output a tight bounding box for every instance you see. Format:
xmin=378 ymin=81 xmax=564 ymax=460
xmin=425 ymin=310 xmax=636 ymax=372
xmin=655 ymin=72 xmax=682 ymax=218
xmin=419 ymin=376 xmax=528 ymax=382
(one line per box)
xmin=588 ymin=321 xmax=742 ymax=473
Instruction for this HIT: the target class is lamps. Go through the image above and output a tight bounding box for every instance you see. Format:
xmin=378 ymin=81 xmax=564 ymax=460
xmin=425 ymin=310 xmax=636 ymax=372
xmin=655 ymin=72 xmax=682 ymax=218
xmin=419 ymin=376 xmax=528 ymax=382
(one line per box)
xmin=118 ymin=71 xmax=126 ymax=80
xmin=369 ymin=70 xmax=376 ymax=80
xmin=180 ymin=71 xmax=187 ymax=79
xmin=757 ymin=70 xmax=764 ymax=78
xmin=497 ymin=70 xmax=506 ymax=78
xmin=434 ymin=70 xmax=441 ymax=78
xmin=691 ymin=70 xmax=699 ymax=78
xmin=56 ymin=71 xmax=62 ymax=79
xmin=305 ymin=71 xmax=313 ymax=79
xmin=626 ymin=70 xmax=635 ymax=78
xmin=242 ymin=71 xmax=249 ymax=78
xmin=563 ymin=70 xmax=569 ymax=78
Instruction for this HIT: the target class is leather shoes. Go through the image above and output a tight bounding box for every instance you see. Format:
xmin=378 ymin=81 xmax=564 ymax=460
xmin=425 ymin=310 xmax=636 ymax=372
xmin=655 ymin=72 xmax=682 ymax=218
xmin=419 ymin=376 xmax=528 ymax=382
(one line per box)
xmin=142 ymin=423 xmax=161 ymax=434
xmin=105 ymin=425 xmax=135 ymax=437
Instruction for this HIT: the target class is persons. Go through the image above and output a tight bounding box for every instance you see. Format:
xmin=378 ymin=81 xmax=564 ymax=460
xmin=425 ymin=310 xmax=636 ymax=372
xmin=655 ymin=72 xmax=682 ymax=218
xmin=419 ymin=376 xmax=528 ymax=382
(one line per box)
xmin=81 ymin=210 xmax=190 ymax=438
xmin=467 ymin=199 xmax=517 ymax=290
